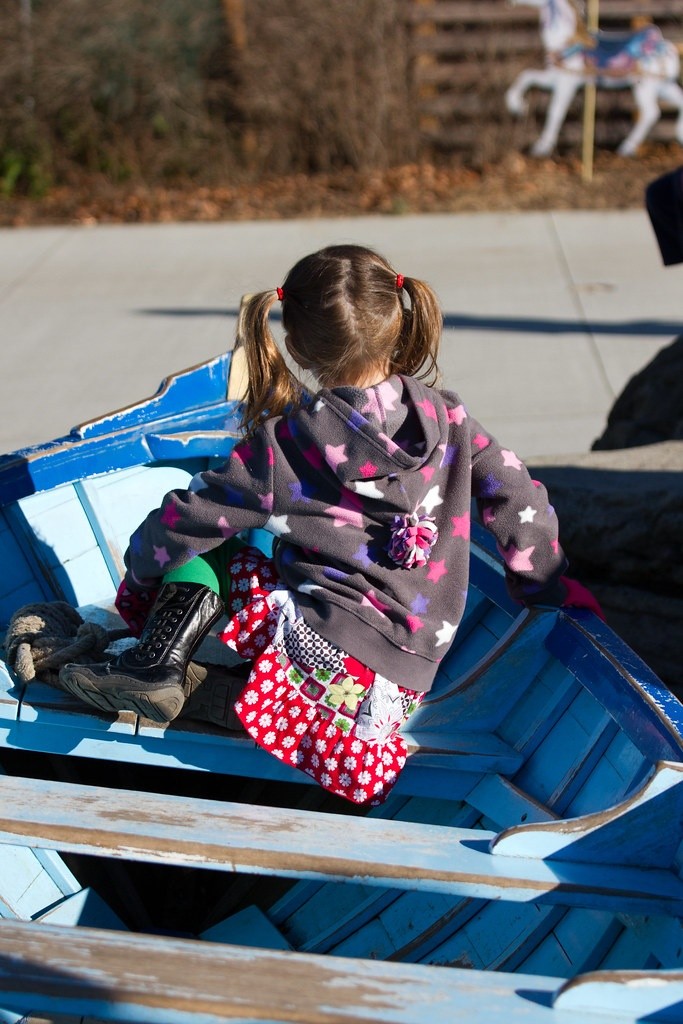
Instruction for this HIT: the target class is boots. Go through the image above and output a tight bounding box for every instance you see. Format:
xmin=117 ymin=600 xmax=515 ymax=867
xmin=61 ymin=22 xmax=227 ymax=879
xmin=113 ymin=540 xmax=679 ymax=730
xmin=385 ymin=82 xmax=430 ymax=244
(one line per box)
xmin=180 ymin=658 xmax=257 ymax=736
xmin=60 ymin=582 xmax=224 ymax=724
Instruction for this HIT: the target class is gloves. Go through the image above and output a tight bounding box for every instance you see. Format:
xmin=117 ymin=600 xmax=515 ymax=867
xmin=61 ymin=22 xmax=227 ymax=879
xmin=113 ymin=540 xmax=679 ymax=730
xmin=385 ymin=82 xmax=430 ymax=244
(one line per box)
xmin=556 ymin=573 xmax=607 ymax=622
xmin=115 ymin=580 xmax=160 ymax=639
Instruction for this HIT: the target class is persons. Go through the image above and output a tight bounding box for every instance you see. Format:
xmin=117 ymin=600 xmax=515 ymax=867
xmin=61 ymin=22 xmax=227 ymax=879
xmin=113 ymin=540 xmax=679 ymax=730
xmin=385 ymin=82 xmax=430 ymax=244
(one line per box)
xmin=58 ymin=244 xmax=605 ymax=807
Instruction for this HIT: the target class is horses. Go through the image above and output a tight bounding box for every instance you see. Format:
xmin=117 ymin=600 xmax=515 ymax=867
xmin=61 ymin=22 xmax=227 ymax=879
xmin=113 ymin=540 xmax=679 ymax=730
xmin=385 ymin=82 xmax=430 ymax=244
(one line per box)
xmin=504 ymin=0 xmax=683 ymax=157
